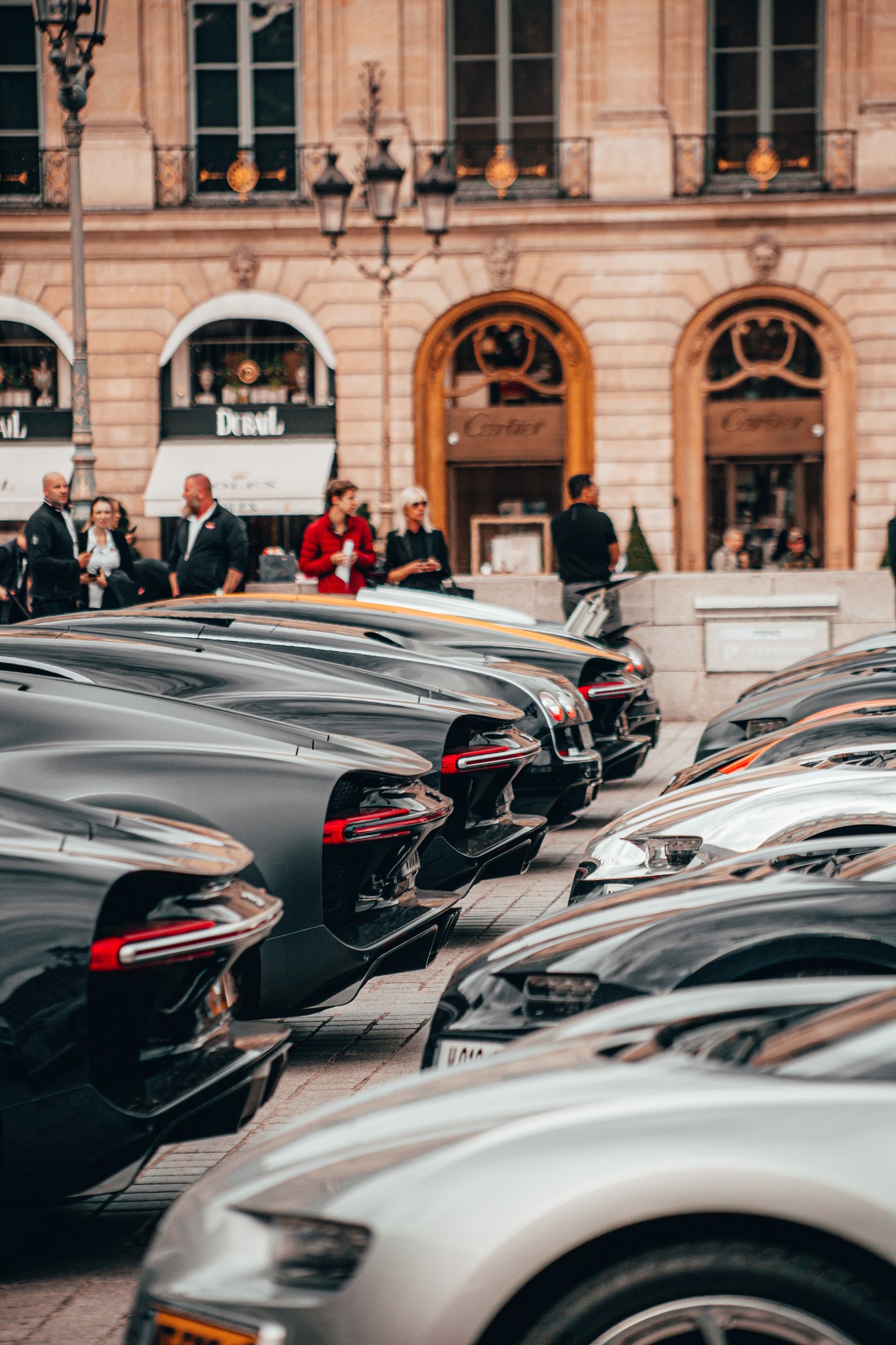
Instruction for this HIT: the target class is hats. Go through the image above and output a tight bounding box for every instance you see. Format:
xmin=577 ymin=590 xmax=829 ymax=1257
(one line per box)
xmin=787 ymin=530 xmax=804 ymax=543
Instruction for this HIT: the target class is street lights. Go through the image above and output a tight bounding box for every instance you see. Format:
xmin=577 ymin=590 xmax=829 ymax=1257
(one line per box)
xmin=315 ymin=144 xmax=458 ymax=558
xmin=32 ymin=0 xmax=109 ymax=550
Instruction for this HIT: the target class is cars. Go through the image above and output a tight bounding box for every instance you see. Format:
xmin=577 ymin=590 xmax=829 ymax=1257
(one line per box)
xmin=1 ymin=588 xmax=896 ymax=1345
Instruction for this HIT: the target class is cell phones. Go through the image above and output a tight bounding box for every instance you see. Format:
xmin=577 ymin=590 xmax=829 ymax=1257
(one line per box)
xmin=90 ymin=545 xmax=96 ymax=553
xmin=130 ymin=526 xmax=137 ymax=534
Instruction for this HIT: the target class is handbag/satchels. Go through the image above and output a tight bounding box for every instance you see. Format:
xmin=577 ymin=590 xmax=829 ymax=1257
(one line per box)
xmin=435 ymin=567 xmax=474 ymax=599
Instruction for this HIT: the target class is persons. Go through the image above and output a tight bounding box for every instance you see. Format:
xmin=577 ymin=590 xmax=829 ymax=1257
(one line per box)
xmin=299 ymin=479 xmax=376 ymax=596
xmin=549 ymin=473 xmax=624 ymax=646
xmin=23 ymin=472 xmax=91 ymax=618
xmin=167 ymin=473 xmax=248 ymax=599
xmin=0 ymin=521 xmax=27 ymax=624
xmin=77 ymin=496 xmax=149 ymax=611
xmin=781 ymin=529 xmax=816 ymax=570
xmin=384 ymin=486 xmax=452 ymax=594
xmin=711 ymin=526 xmax=744 ymax=571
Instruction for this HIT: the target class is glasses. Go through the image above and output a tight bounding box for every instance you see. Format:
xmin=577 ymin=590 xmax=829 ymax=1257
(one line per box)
xmin=411 ymin=501 xmax=427 ymax=509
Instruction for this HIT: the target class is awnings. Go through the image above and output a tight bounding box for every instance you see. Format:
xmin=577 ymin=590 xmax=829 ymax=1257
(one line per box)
xmin=140 ymin=440 xmax=337 ymax=517
xmin=0 ymin=445 xmax=76 ymax=521
